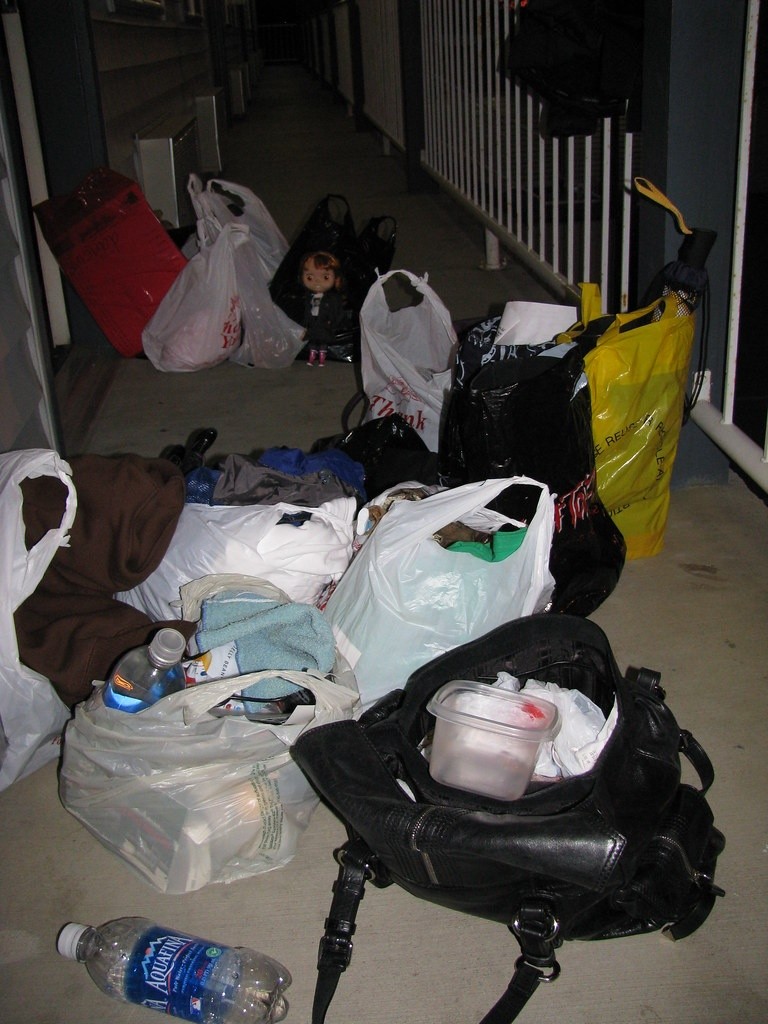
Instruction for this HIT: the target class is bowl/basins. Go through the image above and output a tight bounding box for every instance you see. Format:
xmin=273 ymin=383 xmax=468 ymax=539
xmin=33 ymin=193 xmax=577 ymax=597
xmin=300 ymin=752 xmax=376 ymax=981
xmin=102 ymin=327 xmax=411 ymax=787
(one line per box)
xmin=427 ymin=680 xmax=563 ymax=801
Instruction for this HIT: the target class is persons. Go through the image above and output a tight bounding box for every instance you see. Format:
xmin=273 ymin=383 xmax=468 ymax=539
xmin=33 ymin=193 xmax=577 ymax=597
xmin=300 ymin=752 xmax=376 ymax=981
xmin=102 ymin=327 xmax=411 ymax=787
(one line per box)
xmin=300 ymin=251 xmax=345 ymax=366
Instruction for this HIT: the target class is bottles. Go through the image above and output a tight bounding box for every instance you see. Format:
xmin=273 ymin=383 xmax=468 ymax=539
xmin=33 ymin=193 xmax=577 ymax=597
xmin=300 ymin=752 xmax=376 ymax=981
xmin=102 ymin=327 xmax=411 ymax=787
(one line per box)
xmin=103 ymin=627 xmax=187 ymax=713
xmin=166 ymin=427 xmax=218 ymax=477
xmin=57 ymin=916 xmax=292 ymax=1024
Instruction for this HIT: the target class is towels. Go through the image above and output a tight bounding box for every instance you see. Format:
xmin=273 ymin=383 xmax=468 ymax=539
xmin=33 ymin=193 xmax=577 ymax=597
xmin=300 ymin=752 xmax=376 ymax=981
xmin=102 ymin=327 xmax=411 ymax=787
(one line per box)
xmin=194 ymin=590 xmax=337 ymax=713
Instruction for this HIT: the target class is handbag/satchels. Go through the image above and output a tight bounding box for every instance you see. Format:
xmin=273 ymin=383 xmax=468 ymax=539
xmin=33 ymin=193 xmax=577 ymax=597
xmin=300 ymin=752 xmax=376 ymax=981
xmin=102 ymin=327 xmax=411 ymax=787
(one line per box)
xmin=1 ymin=445 xmax=361 ymax=890
xmin=36 ymin=166 xmax=401 ymax=373
xmin=285 ymin=613 xmax=727 ymax=1022
xmin=326 ymin=263 xmax=709 ymax=694
